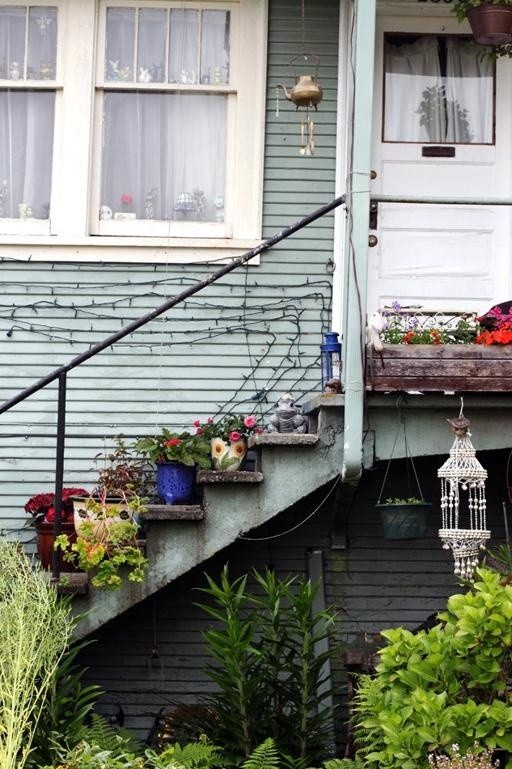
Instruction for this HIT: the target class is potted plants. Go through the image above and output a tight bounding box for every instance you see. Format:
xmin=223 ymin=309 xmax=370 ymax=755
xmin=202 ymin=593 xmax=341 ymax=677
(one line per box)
xmin=54 ymin=484 xmax=150 ymax=591
xmin=376 ymin=497 xmax=432 ymax=538
xmin=449 ymin=0 xmax=512 ymax=63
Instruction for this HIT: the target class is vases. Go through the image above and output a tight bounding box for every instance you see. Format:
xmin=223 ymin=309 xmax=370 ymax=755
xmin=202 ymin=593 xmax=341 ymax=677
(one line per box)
xmin=35 ymin=522 xmax=80 ymax=573
xmin=211 ymin=437 xmax=246 ymax=472
xmin=365 ymin=344 xmax=512 ymax=392
xmin=154 ymin=460 xmax=198 ymax=505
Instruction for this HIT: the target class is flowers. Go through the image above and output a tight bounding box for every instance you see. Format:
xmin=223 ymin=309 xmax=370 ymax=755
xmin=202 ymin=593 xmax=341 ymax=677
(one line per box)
xmin=133 ymin=428 xmax=211 ymax=469
xmin=22 ymin=488 xmax=91 ymax=528
xmin=193 ymin=414 xmax=264 ymax=447
xmin=378 ymin=299 xmax=512 ymax=345
